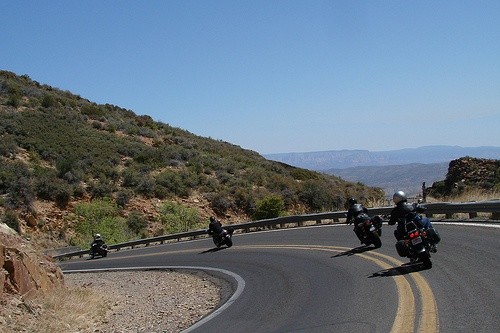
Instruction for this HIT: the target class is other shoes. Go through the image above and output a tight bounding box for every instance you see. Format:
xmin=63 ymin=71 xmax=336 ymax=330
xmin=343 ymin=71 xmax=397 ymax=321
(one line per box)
xmin=433 ymin=244 xmax=438 ymax=248
xmin=361 ymin=242 xmax=364 ymax=244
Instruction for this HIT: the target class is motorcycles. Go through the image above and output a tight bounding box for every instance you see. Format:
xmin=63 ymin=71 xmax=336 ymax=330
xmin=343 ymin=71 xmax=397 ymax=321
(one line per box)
xmin=346 ymin=212 xmax=384 ymax=248
xmin=207 ymin=223 xmax=234 ymax=247
xmin=387 ymin=198 xmax=441 ymax=270
xmin=89 ymin=244 xmax=108 ymax=259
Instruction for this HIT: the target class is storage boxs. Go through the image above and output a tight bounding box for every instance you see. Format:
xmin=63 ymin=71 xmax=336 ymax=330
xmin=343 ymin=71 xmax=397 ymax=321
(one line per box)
xmin=395 ymin=239 xmax=410 ymax=258
xmin=372 ymin=216 xmax=383 ymax=229
xmin=426 ymin=227 xmax=441 ymax=245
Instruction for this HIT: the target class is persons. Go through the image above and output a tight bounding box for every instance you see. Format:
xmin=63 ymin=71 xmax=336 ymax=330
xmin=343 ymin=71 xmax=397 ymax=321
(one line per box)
xmin=346 ymin=197 xmax=376 ymax=244
xmin=92 ymin=233 xmax=105 ymax=254
xmin=207 ymin=216 xmax=225 ymax=244
xmin=388 ymin=190 xmax=439 ymax=258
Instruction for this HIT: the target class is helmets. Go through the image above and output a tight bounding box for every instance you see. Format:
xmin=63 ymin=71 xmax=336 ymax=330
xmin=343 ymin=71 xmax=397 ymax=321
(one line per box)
xmin=347 ymin=198 xmax=357 ymax=207
xmin=210 ymin=216 xmax=215 ymax=222
xmin=96 ymin=234 xmax=101 ymax=239
xmin=393 ymin=191 xmax=407 ymax=205
